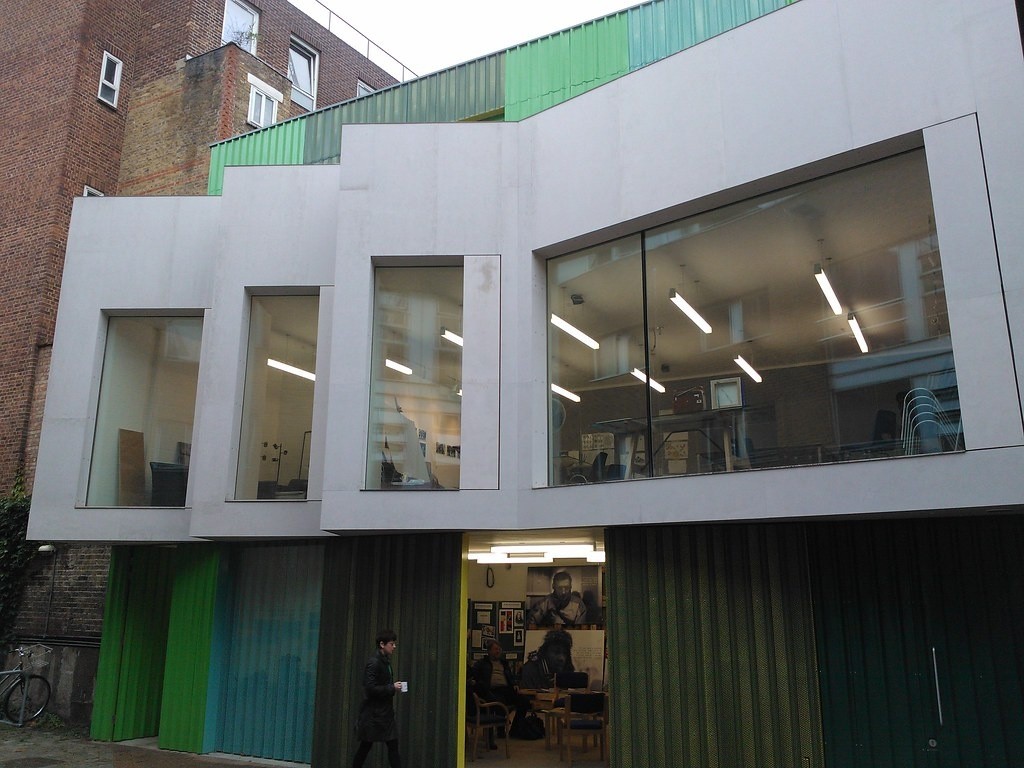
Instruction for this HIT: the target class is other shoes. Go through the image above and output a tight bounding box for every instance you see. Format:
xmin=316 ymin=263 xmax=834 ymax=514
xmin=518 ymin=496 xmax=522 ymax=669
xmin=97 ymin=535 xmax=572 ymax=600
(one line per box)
xmin=490 ymin=745 xmax=497 ymax=750
xmin=497 ymin=730 xmax=506 ymax=738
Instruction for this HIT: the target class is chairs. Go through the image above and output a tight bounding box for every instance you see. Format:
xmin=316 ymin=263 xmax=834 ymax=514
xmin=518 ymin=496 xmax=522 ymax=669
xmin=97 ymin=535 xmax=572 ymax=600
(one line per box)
xmin=466 ymin=691 xmax=511 ymax=761
xmin=558 ymin=693 xmax=612 ymax=768
xmin=864 ymin=408 xmax=898 ymax=460
xmin=587 ymin=451 xmax=608 ymax=481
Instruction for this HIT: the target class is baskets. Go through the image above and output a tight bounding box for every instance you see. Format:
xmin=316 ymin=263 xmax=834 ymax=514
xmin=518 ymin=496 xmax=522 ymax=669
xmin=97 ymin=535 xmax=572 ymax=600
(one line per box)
xmin=23 ymin=644 xmax=54 ymax=669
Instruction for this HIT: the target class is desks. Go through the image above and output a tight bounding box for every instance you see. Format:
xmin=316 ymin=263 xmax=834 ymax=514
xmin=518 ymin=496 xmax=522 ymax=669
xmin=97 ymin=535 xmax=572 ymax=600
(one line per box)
xmin=552 ymin=455 xmax=593 ymax=484
xmin=594 ymin=405 xmax=750 ymax=481
xmin=695 ymin=441 xmax=824 ymax=473
xmin=518 ymin=691 xmax=608 ymax=752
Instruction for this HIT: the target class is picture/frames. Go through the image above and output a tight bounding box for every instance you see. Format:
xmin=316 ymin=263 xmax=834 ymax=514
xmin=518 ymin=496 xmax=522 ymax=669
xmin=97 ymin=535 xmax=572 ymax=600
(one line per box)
xmin=513 ymin=628 xmax=524 ymax=646
xmin=513 ymin=609 xmax=524 ymax=626
xmin=481 ymin=636 xmax=493 ymax=651
xmin=481 ymin=624 xmax=496 ymax=637
xmin=499 ymin=609 xmax=513 ymax=633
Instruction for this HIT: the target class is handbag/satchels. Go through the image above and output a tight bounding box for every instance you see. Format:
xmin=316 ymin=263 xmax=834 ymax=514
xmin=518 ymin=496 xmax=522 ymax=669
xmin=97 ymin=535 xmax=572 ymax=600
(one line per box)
xmin=509 ymin=711 xmax=545 ymax=739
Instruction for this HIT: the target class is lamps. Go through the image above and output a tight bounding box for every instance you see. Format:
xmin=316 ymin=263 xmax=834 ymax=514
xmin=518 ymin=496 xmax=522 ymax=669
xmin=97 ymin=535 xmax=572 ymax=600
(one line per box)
xmin=668 ymin=264 xmax=713 ymax=334
xmin=813 ymin=239 xmax=843 ymax=315
xmin=629 ymin=344 xmax=667 ymax=394
xmin=440 ymin=326 xmax=463 ymax=346
xmin=732 ymin=340 xmax=763 ymax=383
xmin=846 ymin=312 xmax=869 ymax=353
xmin=551 ymin=355 xmax=580 ymax=402
xmin=550 ymin=285 xmax=601 ymax=350
xmin=266 ymin=334 xmax=315 ymax=381
xmin=385 ymin=330 xmax=413 ymax=376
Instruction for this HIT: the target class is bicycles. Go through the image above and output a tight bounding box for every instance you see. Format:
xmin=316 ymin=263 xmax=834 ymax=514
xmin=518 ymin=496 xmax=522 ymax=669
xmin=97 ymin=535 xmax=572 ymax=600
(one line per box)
xmin=0 ymin=640 xmax=53 ymax=729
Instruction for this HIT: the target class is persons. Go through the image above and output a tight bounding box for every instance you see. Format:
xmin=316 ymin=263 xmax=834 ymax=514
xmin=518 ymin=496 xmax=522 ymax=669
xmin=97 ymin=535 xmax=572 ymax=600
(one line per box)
xmin=517 ymin=632 xmax=521 ymax=639
xmin=465 ymin=640 xmax=530 ymax=750
xmin=350 ymin=629 xmax=402 ymax=768
xmin=527 ymin=572 xmax=587 ymax=626
xmin=519 ymin=631 xmax=572 ymax=691
xmin=516 ymin=611 xmax=524 ymax=624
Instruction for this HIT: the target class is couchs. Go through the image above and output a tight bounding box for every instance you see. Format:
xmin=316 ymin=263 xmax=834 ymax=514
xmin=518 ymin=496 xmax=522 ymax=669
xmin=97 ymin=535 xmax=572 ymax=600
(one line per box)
xmin=149 ymin=460 xmax=189 ymax=506
xmin=257 ymin=478 xmax=307 ymax=500
